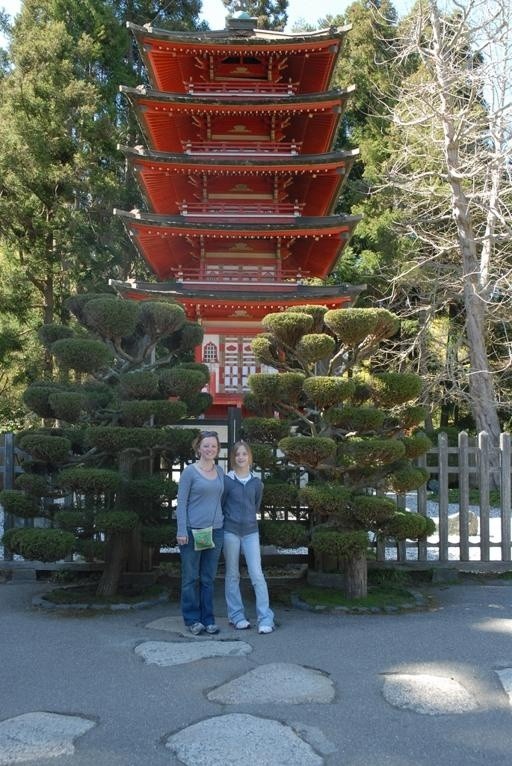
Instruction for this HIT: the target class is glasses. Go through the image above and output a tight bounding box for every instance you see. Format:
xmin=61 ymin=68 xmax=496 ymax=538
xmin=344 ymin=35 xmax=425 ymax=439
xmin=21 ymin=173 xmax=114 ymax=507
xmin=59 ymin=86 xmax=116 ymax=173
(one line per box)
xmin=200 ymin=431 xmax=217 ymax=436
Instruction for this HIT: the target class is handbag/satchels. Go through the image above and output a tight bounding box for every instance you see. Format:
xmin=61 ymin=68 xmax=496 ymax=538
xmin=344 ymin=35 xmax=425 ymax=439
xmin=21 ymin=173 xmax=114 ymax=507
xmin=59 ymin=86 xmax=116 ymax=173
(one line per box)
xmin=192 ymin=526 xmax=215 ymax=551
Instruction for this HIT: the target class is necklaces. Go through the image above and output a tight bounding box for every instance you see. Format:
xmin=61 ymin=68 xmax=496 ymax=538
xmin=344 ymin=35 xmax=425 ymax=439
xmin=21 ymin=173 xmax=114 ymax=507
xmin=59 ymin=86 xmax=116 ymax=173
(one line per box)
xmin=197 ymin=460 xmax=215 ymax=473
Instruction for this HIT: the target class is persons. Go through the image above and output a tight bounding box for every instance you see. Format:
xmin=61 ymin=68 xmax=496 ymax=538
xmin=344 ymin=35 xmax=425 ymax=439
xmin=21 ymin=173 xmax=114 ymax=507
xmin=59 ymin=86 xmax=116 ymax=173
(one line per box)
xmin=175 ymin=429 xmax=225 ymax=637
xmin=221 ymin=439 xmax=276 ymax=635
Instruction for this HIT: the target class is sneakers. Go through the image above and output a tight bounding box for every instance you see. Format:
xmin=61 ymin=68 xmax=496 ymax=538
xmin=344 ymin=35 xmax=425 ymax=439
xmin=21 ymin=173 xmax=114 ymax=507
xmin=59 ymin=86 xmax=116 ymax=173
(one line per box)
xmin=206 ymin=624 xmax=219 ymax=634
xmin=235 ymin=619 xmax=250 ymax=629
xmin=257 ymin=623 xmax=275 ymax=634
xmin=189 ymin=622 xmax=205 ymax=635
